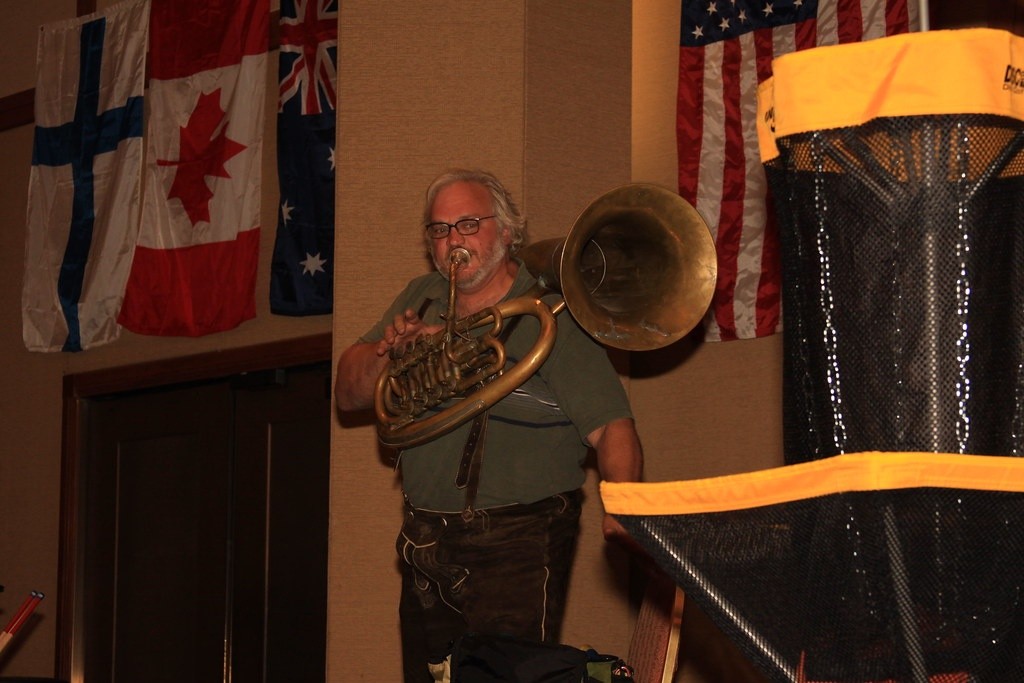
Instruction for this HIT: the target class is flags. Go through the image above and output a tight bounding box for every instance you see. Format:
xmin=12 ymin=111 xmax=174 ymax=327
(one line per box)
xmin=117 ymin=0 xmax=268 ymax=337
xmin=24 ymin=0 xmax=148 ymax=347
xmin=267 ymin=1 xmax=338 ymax=315
xmin=678 ymin=0 xmax=922 ymax=341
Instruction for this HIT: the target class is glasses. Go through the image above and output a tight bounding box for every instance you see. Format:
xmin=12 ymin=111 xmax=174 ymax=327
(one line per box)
xmin=423 ymin=214 xmax=501 ymax=240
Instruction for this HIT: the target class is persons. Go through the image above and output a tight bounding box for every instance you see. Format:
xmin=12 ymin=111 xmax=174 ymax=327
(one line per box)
xmin=342 ymin=170 xmax=643 ymax=683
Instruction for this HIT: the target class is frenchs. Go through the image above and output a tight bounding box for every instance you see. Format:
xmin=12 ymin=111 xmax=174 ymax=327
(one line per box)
xmin=373 ymin=181 xmax=720 ymax=455
